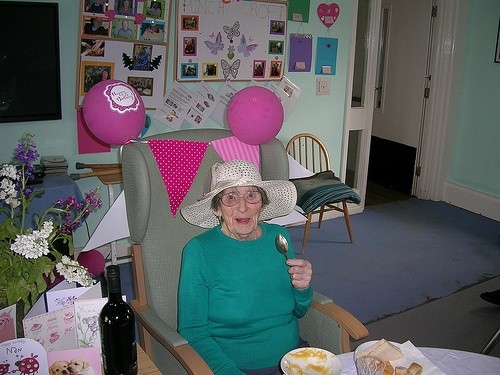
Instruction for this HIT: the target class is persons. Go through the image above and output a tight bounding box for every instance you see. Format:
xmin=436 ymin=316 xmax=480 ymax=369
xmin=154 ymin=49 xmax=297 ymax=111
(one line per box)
xmin=143 ymin=24 xmax=163 ymax=38
xmin=177 ymin=162 xmax=312 ymax=375
xmin=102 ymin=70 xmax=108 ymax=79
xmin=84 ymin=18 xmax=108 ymax=36
xmin=89 ymin=0 xmax=103 ymax=13
xmin=119 ymin=21 xmax=134 ymax=37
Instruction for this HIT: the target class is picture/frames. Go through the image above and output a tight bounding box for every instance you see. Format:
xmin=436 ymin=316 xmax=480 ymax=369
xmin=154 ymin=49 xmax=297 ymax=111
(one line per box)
xmin=76 ymin=0 xmax=173 ymax=110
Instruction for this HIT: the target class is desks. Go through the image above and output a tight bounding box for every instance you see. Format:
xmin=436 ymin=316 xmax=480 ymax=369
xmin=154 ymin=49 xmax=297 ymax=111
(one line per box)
xmin=0 ymin=172 xmax=84 ymax=232
xmin=92 ymin=167 xmax=125 ymax=265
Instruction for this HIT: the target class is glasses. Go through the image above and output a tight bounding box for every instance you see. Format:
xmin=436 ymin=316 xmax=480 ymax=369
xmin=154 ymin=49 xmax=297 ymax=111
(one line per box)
xmin=217 ymin=191 xmax=263 ymax=207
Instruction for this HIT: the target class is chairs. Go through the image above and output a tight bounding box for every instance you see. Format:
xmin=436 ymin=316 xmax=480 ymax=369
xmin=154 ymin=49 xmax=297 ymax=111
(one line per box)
xmin=120 ymin=128 xmax=367 ymax=375
xmin=286 ymin=133 xmax=353 ymax=255
xmin=479 ymin=289 xmax=500 ymax=355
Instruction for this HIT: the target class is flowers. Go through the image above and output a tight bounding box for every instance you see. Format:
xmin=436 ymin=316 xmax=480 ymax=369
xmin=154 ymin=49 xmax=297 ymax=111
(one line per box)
xmin=0 ymin=135 xmax=102 ymax=325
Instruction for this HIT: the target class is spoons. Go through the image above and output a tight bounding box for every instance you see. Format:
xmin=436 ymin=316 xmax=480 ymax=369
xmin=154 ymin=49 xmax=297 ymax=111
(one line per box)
xmin=275 ymin=234 xmax=289 ymax=260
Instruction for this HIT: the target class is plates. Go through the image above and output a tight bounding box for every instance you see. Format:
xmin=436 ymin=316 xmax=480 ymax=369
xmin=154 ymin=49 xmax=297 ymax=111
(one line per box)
xmin=279 ymin=347 xmax=343 ymax=375
xmin=354 ymin=340 xmax=403 ymax=362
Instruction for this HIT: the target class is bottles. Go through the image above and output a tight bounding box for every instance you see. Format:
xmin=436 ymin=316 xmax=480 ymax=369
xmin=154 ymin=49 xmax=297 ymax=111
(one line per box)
xmin=97 ymin=265 xmax=138 ymax=375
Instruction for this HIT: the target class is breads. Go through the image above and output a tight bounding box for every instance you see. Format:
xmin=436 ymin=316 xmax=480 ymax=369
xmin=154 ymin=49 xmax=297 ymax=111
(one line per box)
xmin=373 ymin=360 xmax=423 ymax=375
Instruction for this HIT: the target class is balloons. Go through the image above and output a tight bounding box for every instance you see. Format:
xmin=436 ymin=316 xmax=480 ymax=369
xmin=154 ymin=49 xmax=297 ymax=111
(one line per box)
xmin=227 ymin=86 xmax=284 ymax=145
xmin=83 ymin=79 xmax=146 ymax=145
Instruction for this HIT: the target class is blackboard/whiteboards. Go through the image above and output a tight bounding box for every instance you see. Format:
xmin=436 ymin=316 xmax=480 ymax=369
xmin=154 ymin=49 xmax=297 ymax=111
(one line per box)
xmin=173 ymin=0 xmax=288 ymax=83
xmin=76 ymin=36 xmax=169 ymax=110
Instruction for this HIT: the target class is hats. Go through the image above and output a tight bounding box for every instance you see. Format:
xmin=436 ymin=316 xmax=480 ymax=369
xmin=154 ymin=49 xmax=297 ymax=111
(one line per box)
xmin=180 ymin=160 xmax=297 ymax=229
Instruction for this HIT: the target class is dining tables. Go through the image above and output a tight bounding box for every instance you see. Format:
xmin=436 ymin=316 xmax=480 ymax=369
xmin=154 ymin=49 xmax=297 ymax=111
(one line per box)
xmin=338 ymin=347 xmax=500 ymax=375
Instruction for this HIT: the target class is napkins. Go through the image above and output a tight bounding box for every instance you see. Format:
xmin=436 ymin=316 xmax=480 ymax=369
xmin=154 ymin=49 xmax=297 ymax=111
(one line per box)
xmin=387 ymin=340 xmax=446 ymax=375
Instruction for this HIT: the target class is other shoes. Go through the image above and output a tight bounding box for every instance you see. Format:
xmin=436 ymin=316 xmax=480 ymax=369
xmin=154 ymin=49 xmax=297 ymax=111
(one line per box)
xmin=479 ymin=289 xmax=500 ymax=306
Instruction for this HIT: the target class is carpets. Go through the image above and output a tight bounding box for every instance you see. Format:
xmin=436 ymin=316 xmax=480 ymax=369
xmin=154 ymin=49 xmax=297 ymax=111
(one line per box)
xmin=117 ymin=196 xmax=500 ymax=348
